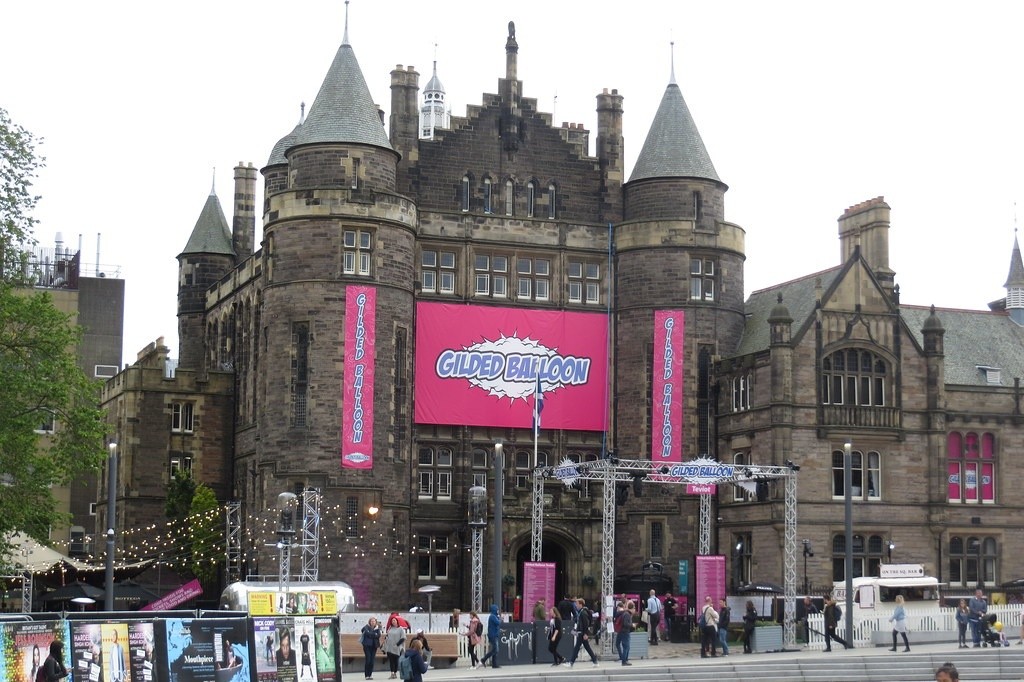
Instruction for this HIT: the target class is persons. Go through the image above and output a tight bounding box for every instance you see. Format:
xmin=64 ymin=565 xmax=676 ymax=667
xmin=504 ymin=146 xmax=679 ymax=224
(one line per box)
xmin=741 ymin=599 xmax=757 ymax=654
xmin=404 ymin=639 xmax=428 ymax=682
xmin=954 ymin=598 xmax=972 ymax=649
xmin=699 ymin=597 xmax=720 ymax=658
xmin=381 ymin=616 xmax=408 ymax=680
xmin=988 ymin=622 xmax=1009 ymax=644
xmin=969 ymin=587 xmax=988 ymax=647
xmin=410 ymin=632 xmax=435 ymax=671
xmin=886 ymin=593 xmax=912 ymax=653
xmin=37 ymin=641 xmax=71 ymax=682
xmin=803 ymin=596 xmax=819 ymax=644
xmin=645 ymin=588 xmax=662 ymax=646
xmin=933 ymin=660 xmax=964 ymax=682
xmin=533 ymin=597 xmax=547 ymax=622
xmin=547 ymin=607 xmax=567 ymax=667
xmin=822 ymin=593 xmax=849 ymax=652
xmin=465 ymin=607 xmax=481 ymax=670
xmin=276 ymin=627 xmax=296 ymax=667
xmin=663 ymin=591 xmax=680 ymax=642
xmin=481 ymin=601 xmax=505 ymax=670
xmin=359 ymin=616 xmax=382 ymax=680
xmin=556 ymin=593 xmax=601 ymax=665
xmin=718 ymin=599 xmax=732 ymax=656
xmin=614 ymin=592 xmax=638 ymax=666
xmin=1015 ymin=611 xmax=1024 ymax=646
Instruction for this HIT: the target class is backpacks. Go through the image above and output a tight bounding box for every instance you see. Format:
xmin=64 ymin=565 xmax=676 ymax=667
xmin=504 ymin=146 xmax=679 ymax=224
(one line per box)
xmin=697 ymin=606 xmax=710 ymax=634
xmin=614 ymin=613 xmax=622 ymax=633
xmin=399 ymin=653 xmax=414 ymax=681
xmin=474 ymin=619 xmax=483 ymax=636
xmin=833 ymin=604 xmax=842 ymax=621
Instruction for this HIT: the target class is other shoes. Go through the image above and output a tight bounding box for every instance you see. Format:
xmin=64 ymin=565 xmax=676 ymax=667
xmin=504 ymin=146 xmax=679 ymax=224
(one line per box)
xmin=823 ymin=648 xmax=831 ymax=651
xmin=651 ymin=642 xmax=658 ymax=645
xmin=843 ymin=642 xmax=847 ymax=649
xmin=564 ymin=663 xmax=573 ymax=667
xmin=366 ymin=677 xmax=369 ymax=680
xmin=700 ymin=652 xmax=728 ymax=658
xmin=481 ymin=658 xmax=486 ymax=668
xmin=492 ymin=665 xmax=501 ymax=669
xmin=593 ymin=659 xmax=598 ymax=666
xmin=559 ymin=658 xmax=565 ymax=664
xmin=888 ymin=647 xmax=897 ymax=650
xmin=903 ymin=648 xmax=910 ymax=652
xmin=369 ymin=675 xmax=373 ymax=680
xmin=958 ymin=640 xmax=1022 ymax=649
xmin=622 ymin=661 xmax=632 ymax=666
xmin=551 ymin=662 xmax=559 ymax=666
xmin=391 ymin=672 xmax=397 ymax=678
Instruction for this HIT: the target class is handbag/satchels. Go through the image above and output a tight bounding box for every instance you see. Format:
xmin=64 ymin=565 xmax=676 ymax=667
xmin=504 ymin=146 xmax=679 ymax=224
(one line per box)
xmin=358 ymin=631 xmax=365 ymax=644
xmin=640 ymin=610 xmax=648 ymax=623
xmin=36 ymin=666 xmax=45 ymax=682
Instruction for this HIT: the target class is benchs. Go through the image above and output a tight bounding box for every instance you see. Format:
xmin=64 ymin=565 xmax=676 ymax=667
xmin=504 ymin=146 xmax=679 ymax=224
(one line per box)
xmin=339 ymin=633 xmax=462 ymax=664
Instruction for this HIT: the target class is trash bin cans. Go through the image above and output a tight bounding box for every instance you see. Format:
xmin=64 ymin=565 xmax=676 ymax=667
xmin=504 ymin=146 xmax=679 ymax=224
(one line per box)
xmin=669 ymin=614 xmax=691 ymax=643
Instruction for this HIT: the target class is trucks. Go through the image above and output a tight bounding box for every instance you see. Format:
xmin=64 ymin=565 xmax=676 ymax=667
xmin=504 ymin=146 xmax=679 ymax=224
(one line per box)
xmin=829 ymin=575 xmax=944 ymax=639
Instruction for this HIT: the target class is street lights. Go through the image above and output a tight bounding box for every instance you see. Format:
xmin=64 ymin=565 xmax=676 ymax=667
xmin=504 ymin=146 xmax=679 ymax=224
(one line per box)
xmin=418 ymin=585 xmax=442 ymax=633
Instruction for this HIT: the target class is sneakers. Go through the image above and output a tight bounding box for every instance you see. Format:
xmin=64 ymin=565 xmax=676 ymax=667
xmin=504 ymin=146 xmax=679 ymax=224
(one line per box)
xmin=476 ymin=661 xmax=482 ymax=669
xmin=468 ymin=666 xmax=476 ymax=670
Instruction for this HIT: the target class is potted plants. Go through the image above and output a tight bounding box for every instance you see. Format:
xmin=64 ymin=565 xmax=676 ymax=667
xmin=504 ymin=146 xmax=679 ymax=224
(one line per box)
xmin=749 ymin=620 xmax=783 ymax=653
